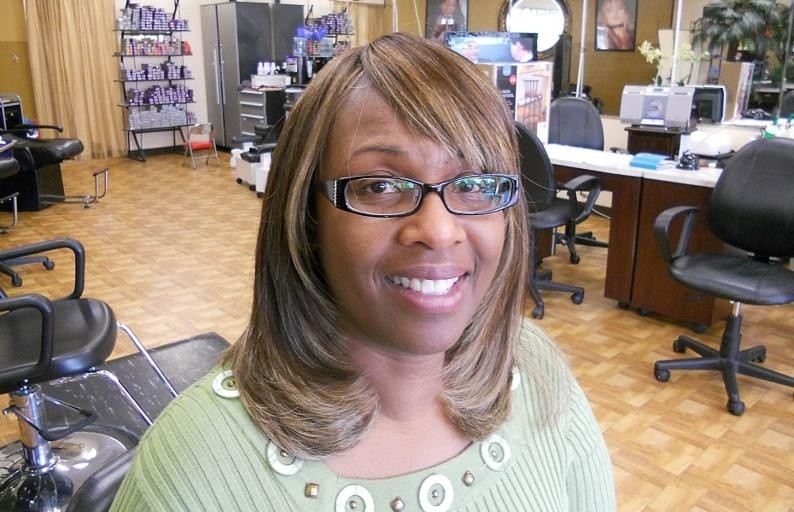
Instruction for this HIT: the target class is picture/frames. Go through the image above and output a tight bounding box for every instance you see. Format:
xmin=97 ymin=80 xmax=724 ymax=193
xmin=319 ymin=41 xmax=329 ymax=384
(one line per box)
xmin=424 ymin=0 xmax=468 ymax=40
xmin=594 ymin=0 xmax=638 ymax=52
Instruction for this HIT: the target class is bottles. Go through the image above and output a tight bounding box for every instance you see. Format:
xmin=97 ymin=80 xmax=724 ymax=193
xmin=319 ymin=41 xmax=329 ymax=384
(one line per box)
xmin=282 ymin=62 xmax=287 ymax=70
xmin=257 ymin=62 xmax=280 ymax=75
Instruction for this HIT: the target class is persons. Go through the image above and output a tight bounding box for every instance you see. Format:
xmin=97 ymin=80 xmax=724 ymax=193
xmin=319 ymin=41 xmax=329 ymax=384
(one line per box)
xmin=599 ymin=0 xmax=635 ymax=50
xmin=106 ymin=33 xmax=616 ymax=512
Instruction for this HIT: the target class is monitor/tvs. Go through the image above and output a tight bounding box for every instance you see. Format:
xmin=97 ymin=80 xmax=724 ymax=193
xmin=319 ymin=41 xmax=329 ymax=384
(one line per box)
xmin=552 ymin=30 xmax=573 ymax=102
xmin=619 ymin=85 xmax=695 ymax=131
xmin=444 ymin=31 xmax=538 ymax=63
xmin=686 ymin=84 xmax=727 ymax=125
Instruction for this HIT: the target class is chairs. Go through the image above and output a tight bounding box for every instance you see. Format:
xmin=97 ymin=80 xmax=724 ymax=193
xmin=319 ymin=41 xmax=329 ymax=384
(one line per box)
xmin=653 ymin=139 xmax=792 ymax=414
xmin=508 ymin=98 xmax=606 ymax=317
xmin=1 ymin=247 xmax=142 ymax=508
xmin=183 ymin=122 xmax=220 ymax=170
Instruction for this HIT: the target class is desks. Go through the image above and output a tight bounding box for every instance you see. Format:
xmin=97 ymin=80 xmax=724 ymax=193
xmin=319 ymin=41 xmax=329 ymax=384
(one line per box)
xmin=544 ymin=136 xmax=723 ymax=334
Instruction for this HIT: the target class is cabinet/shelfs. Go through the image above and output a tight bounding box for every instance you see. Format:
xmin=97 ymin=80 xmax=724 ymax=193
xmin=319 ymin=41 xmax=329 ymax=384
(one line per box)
xmin=112 ymin=1 xmax=196 ymax=163
xmin=283 ymin=29 xmax=358 ymax=87
xmin=237 ymin=87 xmax=282 ymax=139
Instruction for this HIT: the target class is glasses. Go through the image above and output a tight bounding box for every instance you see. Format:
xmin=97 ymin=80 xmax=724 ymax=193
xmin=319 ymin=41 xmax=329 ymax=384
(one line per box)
xmin=314 ymin=174 xmax=520 ymax=217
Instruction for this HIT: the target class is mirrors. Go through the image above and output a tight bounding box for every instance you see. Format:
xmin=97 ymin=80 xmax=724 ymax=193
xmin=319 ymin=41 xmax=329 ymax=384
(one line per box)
xmin=497 ymin=0 xmax=573 ymax=60
